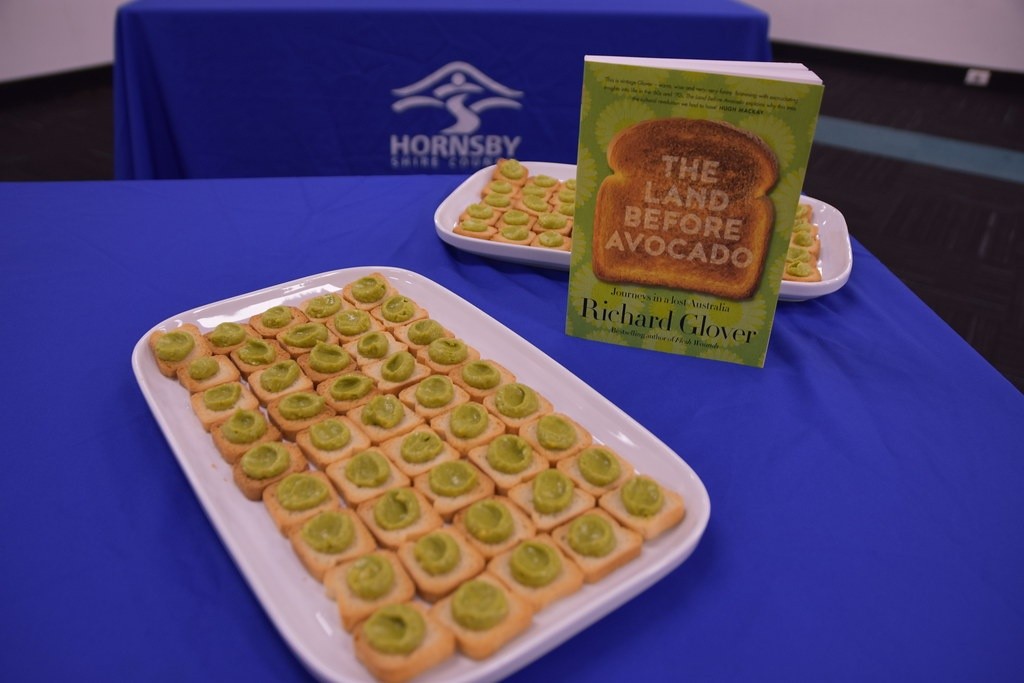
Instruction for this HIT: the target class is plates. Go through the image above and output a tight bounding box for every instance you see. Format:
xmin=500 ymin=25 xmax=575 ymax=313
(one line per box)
xmin=434 ymin=161 xmax=851 ymax=302
xmin=131 ymin=266 xmax=711 ymax=683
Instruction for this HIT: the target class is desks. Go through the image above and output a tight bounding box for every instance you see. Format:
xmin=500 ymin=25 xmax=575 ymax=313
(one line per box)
xmin=1 ymin=173 xmax=1022 ymax=683
xmin=116 ymin=1 xmax=767 ymax=179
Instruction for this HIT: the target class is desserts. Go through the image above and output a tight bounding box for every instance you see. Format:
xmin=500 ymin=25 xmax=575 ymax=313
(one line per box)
xmin=452 ymin=158 xmax=576 ymax=253
xmin=782 ymin=203 xmax=821 ymax=282
xmin=148 ymin=271 xmax=686 ymax=683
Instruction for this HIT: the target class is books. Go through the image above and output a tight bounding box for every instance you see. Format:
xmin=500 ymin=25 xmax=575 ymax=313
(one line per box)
xmin=563 ymin=54 xmax=826 ymax=368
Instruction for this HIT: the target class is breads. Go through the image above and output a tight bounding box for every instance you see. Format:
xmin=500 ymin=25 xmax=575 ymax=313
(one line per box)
xmin=592 ymin=117 xmax=779 ymax=301
